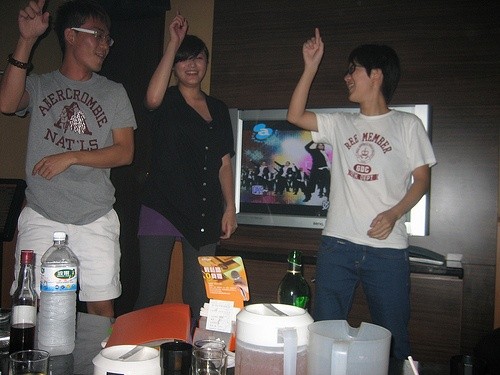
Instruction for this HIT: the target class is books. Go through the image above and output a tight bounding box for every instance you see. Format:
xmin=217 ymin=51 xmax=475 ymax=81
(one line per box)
xmin=104 ymin=254 xmax=250 ymax=368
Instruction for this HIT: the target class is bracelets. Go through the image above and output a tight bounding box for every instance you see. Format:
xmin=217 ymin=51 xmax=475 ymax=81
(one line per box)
xmin=8 ymin=52 xmax=33 ymax=72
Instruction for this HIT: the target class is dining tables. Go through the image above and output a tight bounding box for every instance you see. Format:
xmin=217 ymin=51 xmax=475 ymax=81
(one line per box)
xmin=0 ymin=308 xmax=113 ymax=375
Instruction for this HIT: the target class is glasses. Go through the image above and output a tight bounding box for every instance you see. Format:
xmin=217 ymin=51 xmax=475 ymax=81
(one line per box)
xmin=343 ymin=62 xmax=367 ymax=75
xmin=70 ymin=27 xmax=115 ymax=46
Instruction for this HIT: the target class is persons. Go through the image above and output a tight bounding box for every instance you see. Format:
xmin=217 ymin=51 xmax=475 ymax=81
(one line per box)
xmin=241 ymin=139 xmax=330 ymax=203
xmin=286 ymin=27 xmax=438 ymax=365
xmin=129 ymin=8 xmax=240 ymax=321
xmin=0 ymin=0 xmax=137 ymax=317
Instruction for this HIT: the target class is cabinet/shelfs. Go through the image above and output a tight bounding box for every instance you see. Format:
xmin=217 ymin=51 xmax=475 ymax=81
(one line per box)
xmin=214 ymin=225 xmax=464 ymax=363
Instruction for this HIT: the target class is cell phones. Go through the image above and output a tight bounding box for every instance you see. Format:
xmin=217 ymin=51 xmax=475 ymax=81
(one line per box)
xmin=0 ymin=308 xmax=11 ymax=321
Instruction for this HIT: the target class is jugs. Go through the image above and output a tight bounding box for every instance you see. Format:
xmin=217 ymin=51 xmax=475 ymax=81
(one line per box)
xmin=234 ymin=302 xmax=315 ymax=375
xmin=307 ymin=318 xmax=393 ymax=375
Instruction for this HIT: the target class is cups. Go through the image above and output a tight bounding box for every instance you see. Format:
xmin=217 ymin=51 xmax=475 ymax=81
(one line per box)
xmin=196 ymin=349 xmax=228 ymax=375
xmin=159 ymin=341 xmax=194 ymax=375
xmin=193 ymin=339 xmax=227 ymax=352
xmin=9 ymin=350 xmax=50 ymax=375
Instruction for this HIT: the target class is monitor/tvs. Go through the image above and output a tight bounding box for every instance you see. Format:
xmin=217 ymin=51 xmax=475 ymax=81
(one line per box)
xmin=238 ymin=103 xmax=431 ymax=237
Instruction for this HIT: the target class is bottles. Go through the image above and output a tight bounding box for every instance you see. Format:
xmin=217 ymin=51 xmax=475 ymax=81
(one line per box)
xmin=8 ymin=249 xmax=38 ymax=359
xmin=276 ymin=251 xmax=312 ymax=313
xmin=36 ymin=231 xmax=80 ymax=357
